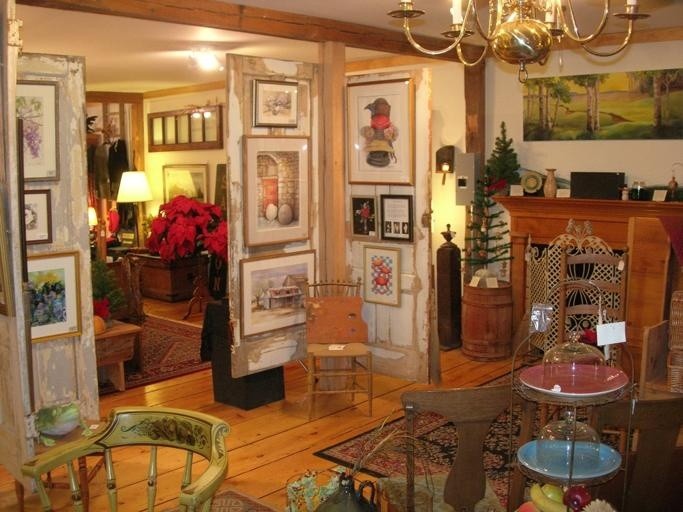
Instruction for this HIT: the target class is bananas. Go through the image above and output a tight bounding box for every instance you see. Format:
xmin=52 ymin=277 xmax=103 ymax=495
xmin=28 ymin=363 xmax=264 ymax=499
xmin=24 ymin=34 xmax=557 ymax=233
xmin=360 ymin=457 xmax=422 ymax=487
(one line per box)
xmin=531 ymin=482 xmax=568 ymax=512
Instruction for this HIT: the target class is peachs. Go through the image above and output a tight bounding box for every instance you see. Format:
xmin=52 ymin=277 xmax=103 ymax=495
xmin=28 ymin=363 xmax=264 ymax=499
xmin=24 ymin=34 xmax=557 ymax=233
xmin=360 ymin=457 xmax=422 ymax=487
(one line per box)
xmin=520 ymin=501 xmax=539 ymax=511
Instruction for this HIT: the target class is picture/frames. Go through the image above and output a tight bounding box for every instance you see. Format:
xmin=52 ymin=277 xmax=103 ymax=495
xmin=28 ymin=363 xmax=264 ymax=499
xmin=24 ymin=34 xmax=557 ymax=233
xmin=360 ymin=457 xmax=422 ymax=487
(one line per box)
xmin=234 ymin=248 xmax=318 ymax=340
xmin=13 ymin=77 xmax=60 ymax=184
xmin=22 ymin=249 xmax=82 ymax=345
xmin=238 ymin=134 xmax=312 ymax=249
xmin=21 ymin=186 xmax=53 ymax=245
xmin=249 ymin=76 xmax=299 ymax=129
xmin=341 ymin=77 xmax=416 ymax=309
xmin=161 ymin=161 xmax=210 ymax=207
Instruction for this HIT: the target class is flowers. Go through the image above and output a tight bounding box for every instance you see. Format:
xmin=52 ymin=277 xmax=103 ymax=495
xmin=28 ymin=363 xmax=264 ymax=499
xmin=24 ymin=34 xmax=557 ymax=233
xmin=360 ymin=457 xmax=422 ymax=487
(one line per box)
xmin=141 ymin=195 xmax=228 ymax=265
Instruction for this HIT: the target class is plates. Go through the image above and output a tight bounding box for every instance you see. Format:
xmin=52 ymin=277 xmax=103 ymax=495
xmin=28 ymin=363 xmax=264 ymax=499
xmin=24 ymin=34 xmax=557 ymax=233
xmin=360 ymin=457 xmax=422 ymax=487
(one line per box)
xmin=520 ymin=172 xmax=542 ymax=194
xmin=515 ymin=438 xmax=623 ymax=484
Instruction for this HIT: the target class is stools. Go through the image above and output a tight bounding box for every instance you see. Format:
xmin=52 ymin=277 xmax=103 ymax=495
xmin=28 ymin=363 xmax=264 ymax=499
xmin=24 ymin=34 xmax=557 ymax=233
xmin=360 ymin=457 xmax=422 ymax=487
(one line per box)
xmin=91 ymin=318 xmax=142 ymax=391
xmin=16 ymin=416 xmax=105 ymax=510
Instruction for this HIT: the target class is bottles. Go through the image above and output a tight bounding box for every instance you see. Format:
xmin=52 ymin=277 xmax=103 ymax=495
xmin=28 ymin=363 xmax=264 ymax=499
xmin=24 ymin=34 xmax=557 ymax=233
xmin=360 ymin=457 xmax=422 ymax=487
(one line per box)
xmin=668 ymin=176 xmax=678 ymax=200
xmin=631 ymin=181 xmax=638 ymax=200
xmin=639 ymin=181 xmax=647 ymax=200
xmin=621 ymin=183 xmax=629 ymax=201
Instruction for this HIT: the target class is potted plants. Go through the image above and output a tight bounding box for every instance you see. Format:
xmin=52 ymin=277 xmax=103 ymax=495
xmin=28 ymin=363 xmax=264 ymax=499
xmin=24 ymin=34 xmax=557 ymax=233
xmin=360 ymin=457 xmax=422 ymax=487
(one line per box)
xmin=89 ymin=259 xmax=128 ymax=323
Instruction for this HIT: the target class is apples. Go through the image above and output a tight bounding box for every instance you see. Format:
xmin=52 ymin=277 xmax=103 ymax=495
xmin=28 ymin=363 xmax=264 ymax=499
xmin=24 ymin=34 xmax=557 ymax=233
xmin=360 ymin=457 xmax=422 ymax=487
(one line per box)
xmin=541 ymin=484 xmax=561 ymax=502
xmin=563 ymin=486 xmax=591 ymax=512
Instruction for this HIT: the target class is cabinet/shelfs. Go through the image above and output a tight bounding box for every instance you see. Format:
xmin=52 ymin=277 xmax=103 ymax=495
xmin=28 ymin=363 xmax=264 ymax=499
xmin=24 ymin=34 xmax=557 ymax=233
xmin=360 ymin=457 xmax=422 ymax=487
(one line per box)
xmin=145 ymin=105 xmax=223 ymax=153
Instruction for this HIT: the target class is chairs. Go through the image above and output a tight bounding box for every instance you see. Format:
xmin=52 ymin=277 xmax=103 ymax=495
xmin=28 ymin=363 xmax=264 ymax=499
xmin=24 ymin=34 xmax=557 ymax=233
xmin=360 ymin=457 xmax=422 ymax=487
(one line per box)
xmin=582 ymin=389 xmax=681 ymax=508
xmin=395 ymin=382 xmax=538 ymax=510
xmin=558 ymin=244 xmax=629 ymax=350
xmin=21 ymin=405 xmax=229 ymax=511
xmin=636 ymin=291 xmax=682 ymax=399
xmin=300 ymin=275 xmax=374 ymax=421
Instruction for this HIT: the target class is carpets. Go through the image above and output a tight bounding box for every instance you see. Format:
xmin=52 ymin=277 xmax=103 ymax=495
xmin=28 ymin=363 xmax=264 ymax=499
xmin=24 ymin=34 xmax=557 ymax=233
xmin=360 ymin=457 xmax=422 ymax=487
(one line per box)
xmin=311 ymin=358 xmax=636 ymax=508
xmin=98 ymin=314 xmax=212 ymax=401
xmin=163 ymin=481 xmax=279 ymax=511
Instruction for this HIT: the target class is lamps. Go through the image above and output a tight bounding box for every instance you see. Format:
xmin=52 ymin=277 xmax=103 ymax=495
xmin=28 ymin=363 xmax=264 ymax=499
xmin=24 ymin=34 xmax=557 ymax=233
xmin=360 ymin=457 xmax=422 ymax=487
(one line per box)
xmin=384 ymin=0 xmax=653 ymax=86
xmin=115 ymin=170 xmax=153 ymax=247
xmin=87 ymin=207 xmax=98 ymax=249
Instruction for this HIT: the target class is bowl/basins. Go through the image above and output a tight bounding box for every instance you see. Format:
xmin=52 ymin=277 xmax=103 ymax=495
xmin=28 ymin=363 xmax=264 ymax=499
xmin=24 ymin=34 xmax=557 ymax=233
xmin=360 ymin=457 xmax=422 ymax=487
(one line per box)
xmin=518 ymin=365 xmax=631 ymax=402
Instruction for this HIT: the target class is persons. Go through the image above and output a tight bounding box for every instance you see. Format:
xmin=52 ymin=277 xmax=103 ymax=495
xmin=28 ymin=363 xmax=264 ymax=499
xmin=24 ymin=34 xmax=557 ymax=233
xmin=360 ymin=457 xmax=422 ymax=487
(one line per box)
xmin=360 ymin=202 xmax=370 ymax=232
